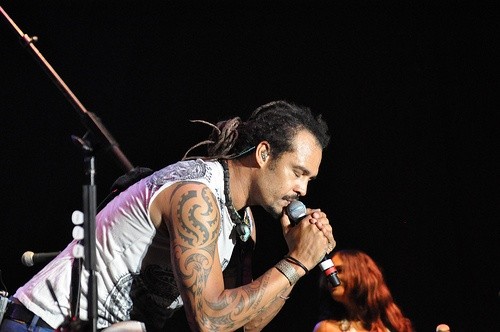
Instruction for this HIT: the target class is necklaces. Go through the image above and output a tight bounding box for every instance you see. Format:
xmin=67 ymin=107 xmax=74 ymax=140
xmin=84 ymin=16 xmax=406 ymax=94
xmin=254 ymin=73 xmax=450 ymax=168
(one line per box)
xmin=219 ymin=156 xmax=252 ymax=242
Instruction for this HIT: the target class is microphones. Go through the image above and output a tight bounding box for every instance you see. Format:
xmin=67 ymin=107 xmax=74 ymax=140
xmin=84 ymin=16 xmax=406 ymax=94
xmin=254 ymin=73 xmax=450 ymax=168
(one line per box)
xmin=286 ymin=201 xmax=341 ymax=286
xmin=21 ymin=251 xmax=62 ymax=267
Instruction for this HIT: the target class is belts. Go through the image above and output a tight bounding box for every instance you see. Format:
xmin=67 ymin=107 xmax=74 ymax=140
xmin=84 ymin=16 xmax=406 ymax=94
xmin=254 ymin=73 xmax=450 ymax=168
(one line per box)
xmin=3 ymin=303 xmax=55 ymax=330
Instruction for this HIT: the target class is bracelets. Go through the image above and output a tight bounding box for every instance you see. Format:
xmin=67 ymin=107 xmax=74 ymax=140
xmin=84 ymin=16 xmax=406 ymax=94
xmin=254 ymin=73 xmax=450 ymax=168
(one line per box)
xmin=279 ymin=294 xmax=290 ymax=300
xmin=276 ymin=259 xmax=300 ymax=285
xmin=284 ymin=254 xmax=310 ymax=274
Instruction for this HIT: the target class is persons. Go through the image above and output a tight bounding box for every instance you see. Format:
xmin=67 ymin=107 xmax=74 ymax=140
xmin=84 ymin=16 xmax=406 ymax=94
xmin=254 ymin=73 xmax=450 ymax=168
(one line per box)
xmin=0 ymin=101 xmax=338 ymax=332
xmin=311 ymin=248 xmax=451 ymax=331
